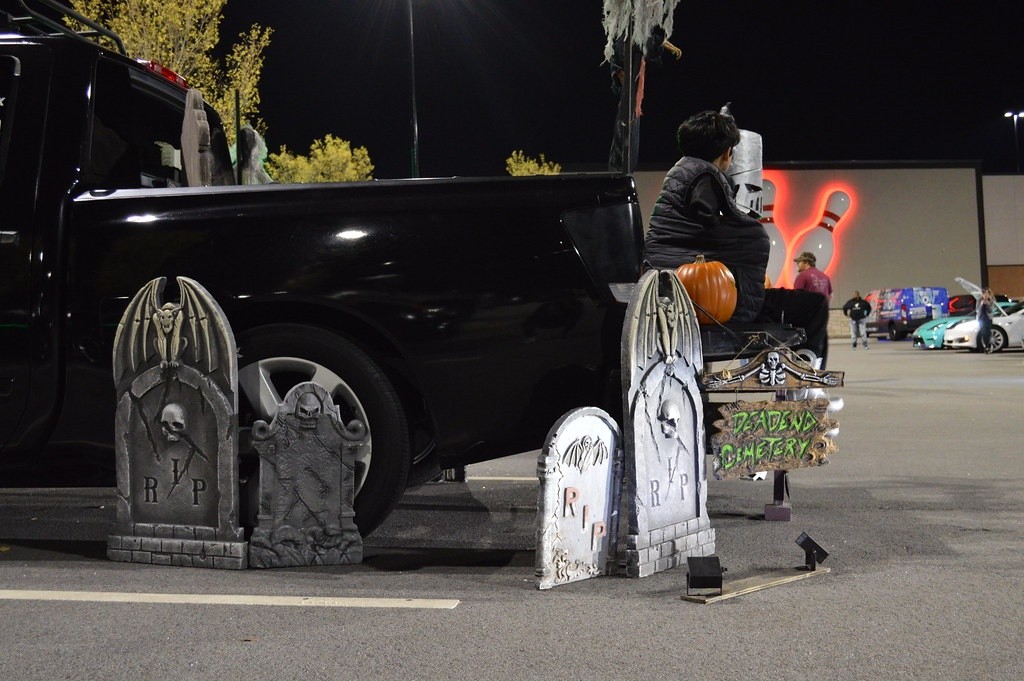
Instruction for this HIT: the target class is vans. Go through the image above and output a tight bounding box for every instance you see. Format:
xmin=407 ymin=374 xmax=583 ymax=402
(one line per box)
xmin=857 ymin=287 xmax=948 ymax=340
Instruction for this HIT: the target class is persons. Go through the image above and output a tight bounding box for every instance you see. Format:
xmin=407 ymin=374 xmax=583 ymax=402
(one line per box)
xmin=976 ymin=288 xmax=995 ymax=354
xmin=792 ymin=252 xmax=832 ymax=369
xmin=644 ymin=110 xmax=769 ymax=324
xmin=842 ymin=292 xmax=871 ymax=350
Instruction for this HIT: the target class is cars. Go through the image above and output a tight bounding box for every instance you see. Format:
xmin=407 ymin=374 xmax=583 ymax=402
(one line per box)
xmin=944 ymin=301 xmax=1024 ymax=353
xmin=911 ymin=302 xmax=1024 ymax=352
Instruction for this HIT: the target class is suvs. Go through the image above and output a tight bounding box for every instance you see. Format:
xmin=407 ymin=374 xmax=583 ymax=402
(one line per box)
xmin=948 ymin=294 xmax=1009 ymax=317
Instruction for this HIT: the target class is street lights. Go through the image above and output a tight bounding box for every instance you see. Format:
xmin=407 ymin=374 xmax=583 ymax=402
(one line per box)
xmin=1003 ymin=111 xmax=1024 ymax=174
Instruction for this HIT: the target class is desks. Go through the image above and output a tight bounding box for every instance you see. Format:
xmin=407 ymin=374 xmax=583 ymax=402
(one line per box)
xmin=698 ymin=322 xmax=806 ymax=520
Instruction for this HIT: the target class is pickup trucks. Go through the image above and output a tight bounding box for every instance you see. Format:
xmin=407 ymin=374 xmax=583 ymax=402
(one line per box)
xmin=0 ymin=0 xmax=653 ymax=551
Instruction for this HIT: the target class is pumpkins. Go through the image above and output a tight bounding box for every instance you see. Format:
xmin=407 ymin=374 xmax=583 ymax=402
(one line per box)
xmin=676 ymin=254 xmax=737 ymax=323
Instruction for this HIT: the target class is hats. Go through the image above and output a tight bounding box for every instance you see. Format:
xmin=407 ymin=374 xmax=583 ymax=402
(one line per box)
xmin=794 ymin=256 xmax=816 ymax=263
